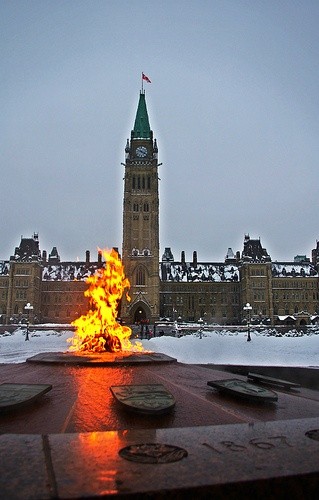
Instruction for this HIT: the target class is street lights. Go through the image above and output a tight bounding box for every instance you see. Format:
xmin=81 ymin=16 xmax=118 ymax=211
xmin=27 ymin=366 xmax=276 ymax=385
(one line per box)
xmin=243 ymin=302 xmax=252 ymax=341
xmin=25 ymin=303 xmax=33 ymax=341
xmin=198 ymin=318 xmax=203 ymax=339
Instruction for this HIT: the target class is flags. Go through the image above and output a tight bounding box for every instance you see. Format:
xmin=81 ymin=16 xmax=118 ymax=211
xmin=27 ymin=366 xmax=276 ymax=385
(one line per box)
xmin=142 ymin=74 xmax=151 ymax=83
